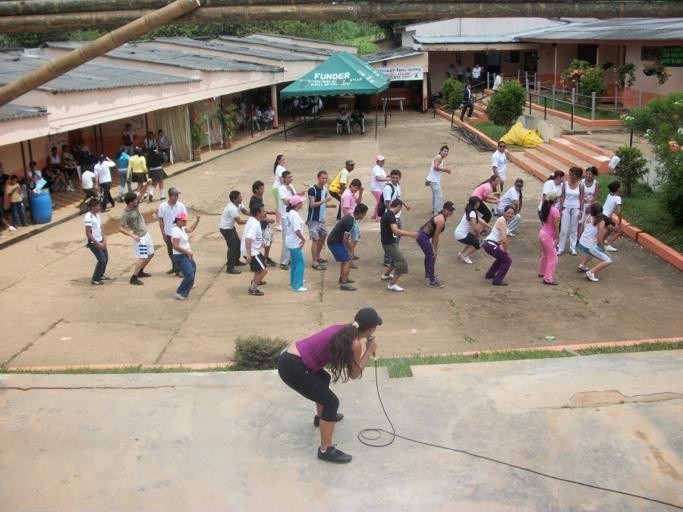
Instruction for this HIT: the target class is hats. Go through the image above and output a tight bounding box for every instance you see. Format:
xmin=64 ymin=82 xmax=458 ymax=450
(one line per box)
xmin=442 ymin=201 xmax=456 ymax=211
xmin=175 ymin=211 xmax=190 ymax=220
xmin=168 ymin=187 xmax=181 ymax=196
xmin=288 ymin=195 xmax=306 ymax=206
xmin=354 ymin=307 xmax=382 ymax=327
xmin=376 ymin=155 xmax=386 ymax=162
xmin=545 ymin=192 xmax=558 ymax=201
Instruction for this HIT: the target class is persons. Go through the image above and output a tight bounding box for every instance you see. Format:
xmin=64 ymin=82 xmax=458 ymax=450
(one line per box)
xmin=338 ymin=108 xmax=365 ymax=135
xmin=305 ymin=171 xmax=337 ymax=270
xmin=327 ymin=203 xmax=368 ymax=290
xmin=293 ymin=95 xmax=324 ymax=116
xmin=525 ymin=53 xmax=542 ymax=88
xmin=538 ymin=166 xmax=623 ymax=285
xmin=370 ymin=155 xmax=420 ymax=293
xmin=454 ymin=141 xmax=523 ymax=286
xmin=232 ymin=96 xmax=276 ymax=133
xmin=278 ymin=308 xmax=382 ymax=464
xmin=157 ymin=187 xmax=188 ymax=277
xmin=271 ymin=154 xmax=308 ymax=291
xmin=416 ymin=201 xmax=457 ymax=289
xmin=329 ymin=159 xmax=357 ymax=219
xmin=85 ymin=199 xmax=111 ymax=285
xmin=425 ymin=145 xmax=451 ymax=217
xmin=1 ymin=123 xmax=172 ymax=229
xmin=171 ymin=210 xmax=200 ymax=300
xmin=449 ymin=61 xmax=504 ymax=122
xmin=118 ymin=181 xmax=155 ymax=286
xmin=219 ymin=180 xmax=277 ymax=296
xmin=341 ymin=179 xmax=364 ymax=269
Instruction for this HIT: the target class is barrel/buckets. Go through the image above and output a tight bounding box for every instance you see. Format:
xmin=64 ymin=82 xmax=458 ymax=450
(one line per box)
xmin=31 ymin=193 xmax=53 ymax=223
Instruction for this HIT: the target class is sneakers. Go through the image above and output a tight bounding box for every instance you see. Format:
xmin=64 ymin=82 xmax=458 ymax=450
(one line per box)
xmin=116 ymin=190 xmax=166 ymax=202
xmin=248 ymin=289 xmax=265 ymax=295
xmin=130 ymin=279 xmax=144 ymax=285
xmin=578 ymin=263 xmax=590 ymax=274
xmin=314 ymin=413 xmax=343 ymax=427
xmin=101 ymin=275 xmax=107 ymax=280
xmin=228 ymin=254 xmax=359 ymax=274
xmin=317 ymin=446 xmax=352 ymax=464
xmin=251 ymin=279 xmax=266 ymax=285
xmin=485 ymin=272 xmax=497 ymax=279
xmin=339 ymin=274 xmax=445 ymax=292
xmin=492 ymin=280 xmax=508 ymax=286
xmin=586 ymin=272 xmax=599 ymax=284
xmin=296 ymin=286 xmax=307 ymax=292
xmin=538 ymin=240 xmax=618 ymax=256
xmin=92 ymin=281 xmax=105 ymax=285
xmin=458 ymin=251 xmax=473 ymax=264
xmin=138 ymin=271 xmax=152 ymax=277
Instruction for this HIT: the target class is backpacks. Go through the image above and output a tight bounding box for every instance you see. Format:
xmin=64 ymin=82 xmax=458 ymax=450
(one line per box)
xmin=376 ymin=183 xmax=395 ymax=217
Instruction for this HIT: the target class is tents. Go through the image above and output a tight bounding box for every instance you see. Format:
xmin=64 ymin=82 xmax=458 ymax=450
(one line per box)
xmin=280 ymin=50 xmax=392 ymax=142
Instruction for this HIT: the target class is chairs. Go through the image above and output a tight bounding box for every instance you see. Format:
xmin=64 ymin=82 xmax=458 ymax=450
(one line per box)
xmin=337 ymin=120 xmax=349 ymax=134
xmin=349 ymin=112 xmax=364 ymax=134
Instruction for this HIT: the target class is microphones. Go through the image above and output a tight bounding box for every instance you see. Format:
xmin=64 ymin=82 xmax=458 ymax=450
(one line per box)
xmin=367 ymin=336 xmax=380 ymax=365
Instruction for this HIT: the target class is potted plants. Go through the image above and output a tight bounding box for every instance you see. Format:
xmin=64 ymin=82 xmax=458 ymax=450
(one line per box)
xmin=190 ymin=105 xmax=204 ymax=161
xmin=216 ymin=103 xmax=244 ymax=148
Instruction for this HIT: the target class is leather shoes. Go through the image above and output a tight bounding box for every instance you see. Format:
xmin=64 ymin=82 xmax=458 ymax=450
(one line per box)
xmin=544 ymin=280 xmax=558 ymax=286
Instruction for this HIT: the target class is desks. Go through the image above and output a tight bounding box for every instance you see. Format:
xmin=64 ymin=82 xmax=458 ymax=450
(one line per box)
xmin=381 ymin=97 xmax=405 ymax=112
